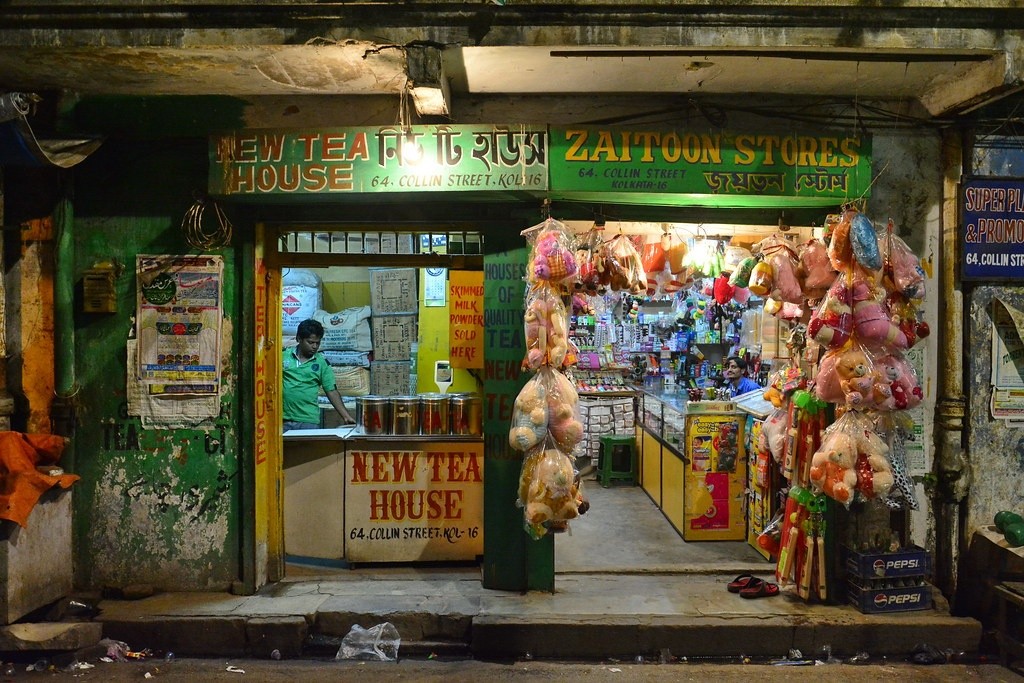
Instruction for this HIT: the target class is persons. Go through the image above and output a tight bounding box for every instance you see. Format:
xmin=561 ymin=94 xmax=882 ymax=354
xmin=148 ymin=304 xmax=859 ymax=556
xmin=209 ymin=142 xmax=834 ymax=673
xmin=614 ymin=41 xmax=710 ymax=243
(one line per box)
xmin=725 ymin=357 xmax=763 ymax=398
xmin=693 ymin=436 xmax=707 ymax=455
xmin=282 ymin=319 xmax=356 ymax=435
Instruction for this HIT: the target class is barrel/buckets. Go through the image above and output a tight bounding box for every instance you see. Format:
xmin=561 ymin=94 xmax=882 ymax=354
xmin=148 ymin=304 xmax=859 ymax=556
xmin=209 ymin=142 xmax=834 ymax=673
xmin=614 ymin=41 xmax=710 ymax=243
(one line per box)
xmin=355 ymin=392 xmax=483 ymax=436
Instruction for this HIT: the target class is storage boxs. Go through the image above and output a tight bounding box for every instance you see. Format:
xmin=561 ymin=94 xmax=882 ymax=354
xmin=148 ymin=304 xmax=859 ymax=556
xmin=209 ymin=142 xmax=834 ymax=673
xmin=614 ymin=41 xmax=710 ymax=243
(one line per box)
xmin=839 ymin=540 xmax=933 ymax=614
xmin=369 ymin=267 xmax=419 ymax=396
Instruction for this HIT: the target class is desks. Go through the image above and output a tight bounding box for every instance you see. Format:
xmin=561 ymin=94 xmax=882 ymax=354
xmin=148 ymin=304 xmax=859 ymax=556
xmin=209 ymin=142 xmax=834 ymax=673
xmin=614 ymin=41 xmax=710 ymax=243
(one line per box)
xmin=281 ymin=428 xmax=484 ymax=572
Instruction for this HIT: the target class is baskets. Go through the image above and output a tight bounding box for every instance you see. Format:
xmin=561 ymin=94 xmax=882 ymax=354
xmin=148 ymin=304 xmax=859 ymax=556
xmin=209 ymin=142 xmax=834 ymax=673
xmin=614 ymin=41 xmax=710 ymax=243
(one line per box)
xmin=832 ymin=540 xmax=935 ymax=615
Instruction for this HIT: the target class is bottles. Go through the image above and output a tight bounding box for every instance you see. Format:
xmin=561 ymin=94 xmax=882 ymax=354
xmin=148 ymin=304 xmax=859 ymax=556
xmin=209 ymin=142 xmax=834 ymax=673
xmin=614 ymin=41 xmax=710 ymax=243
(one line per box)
xmin=848 ymin=572 xmax=925 ymax=590
xmin=848 ymin=531 xmax=917 ymax=556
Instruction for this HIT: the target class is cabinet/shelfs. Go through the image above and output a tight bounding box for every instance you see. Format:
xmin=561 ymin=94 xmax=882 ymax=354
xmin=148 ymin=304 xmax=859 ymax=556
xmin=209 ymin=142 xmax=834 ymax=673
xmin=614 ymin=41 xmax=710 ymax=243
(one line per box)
xmin=634 ymin=384 xmax=748 ymax=542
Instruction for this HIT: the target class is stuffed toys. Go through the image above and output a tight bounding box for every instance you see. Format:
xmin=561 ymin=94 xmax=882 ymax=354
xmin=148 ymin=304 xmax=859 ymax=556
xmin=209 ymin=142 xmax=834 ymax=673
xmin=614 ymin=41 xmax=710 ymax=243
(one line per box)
xmin=765 ymin=213 xmax=930 ymax=510
xmin=507 ymin=221 xmax=595 ymax=540
xmin=570 ymin=234 xmax=803 ymax=305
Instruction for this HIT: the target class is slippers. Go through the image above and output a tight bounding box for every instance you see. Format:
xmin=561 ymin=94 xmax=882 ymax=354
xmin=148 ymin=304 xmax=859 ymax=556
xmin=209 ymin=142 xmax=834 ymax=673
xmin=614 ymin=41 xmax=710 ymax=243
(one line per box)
xmin=727 ymin=570 xmax=756 ymax=593
xmin=741 ymin=578 xmax=779 ymax=599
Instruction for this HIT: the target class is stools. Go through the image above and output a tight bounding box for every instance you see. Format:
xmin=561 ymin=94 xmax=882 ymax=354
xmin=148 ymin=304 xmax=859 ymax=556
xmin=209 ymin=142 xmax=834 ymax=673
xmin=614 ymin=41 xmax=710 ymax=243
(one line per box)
xmin=596 ymin=434 xmax=637 ymax=488
